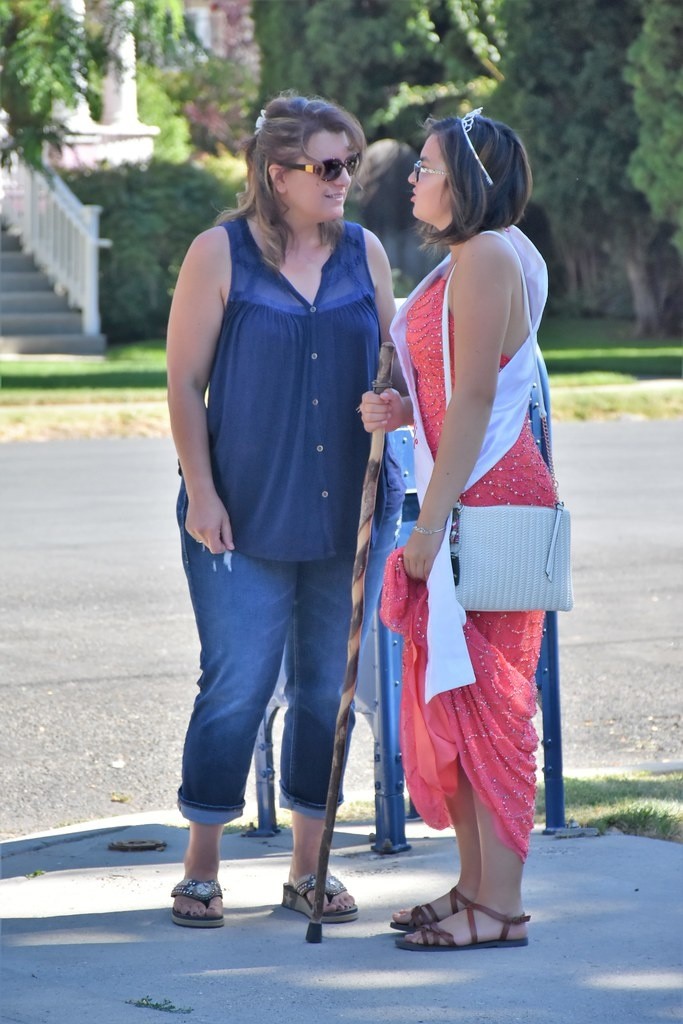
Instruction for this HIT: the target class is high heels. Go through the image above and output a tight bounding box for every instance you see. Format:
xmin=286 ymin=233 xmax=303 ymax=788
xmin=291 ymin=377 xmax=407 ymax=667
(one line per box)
xmin=170 ymin=878 xmax=225 ymax=929
xmin=281 ymin=870 xmax=360 ymax=924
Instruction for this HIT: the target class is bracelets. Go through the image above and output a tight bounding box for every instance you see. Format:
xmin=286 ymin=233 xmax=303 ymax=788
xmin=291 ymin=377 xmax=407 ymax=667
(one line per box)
xmin=413 ymin=521 xmax=447 ymax=533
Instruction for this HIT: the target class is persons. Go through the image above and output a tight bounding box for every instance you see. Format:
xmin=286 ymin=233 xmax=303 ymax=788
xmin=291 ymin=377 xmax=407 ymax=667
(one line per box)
xmin=356 ymin=103 xmax=563 ymax=951
xmin=171 ymin=91 xmax=398 ymax=927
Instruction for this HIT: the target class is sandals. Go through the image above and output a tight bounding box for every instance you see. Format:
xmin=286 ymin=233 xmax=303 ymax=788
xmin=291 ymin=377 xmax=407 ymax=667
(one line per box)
xmin=390 ymin=887 xmax=473 ymax=932
xmin=395 ymin=903 xmax=531 ymax=952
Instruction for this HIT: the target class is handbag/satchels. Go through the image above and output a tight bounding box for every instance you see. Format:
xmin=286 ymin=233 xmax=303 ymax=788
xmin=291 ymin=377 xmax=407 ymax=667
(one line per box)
xmin=453 ymin=502 xmax=573 ymax=611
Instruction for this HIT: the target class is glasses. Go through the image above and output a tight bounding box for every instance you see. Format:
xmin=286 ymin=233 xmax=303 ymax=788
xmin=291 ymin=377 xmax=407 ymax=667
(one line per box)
xmin=284 ymin=152 xmax=360 ymax=182
xmin=414 ymin=160 xmax=448 ymax=182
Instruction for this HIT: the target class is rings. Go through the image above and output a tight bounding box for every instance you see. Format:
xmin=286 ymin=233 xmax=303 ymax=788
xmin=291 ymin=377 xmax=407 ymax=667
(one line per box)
xmin=195 ymin=539 xmax=204 ymax=544
